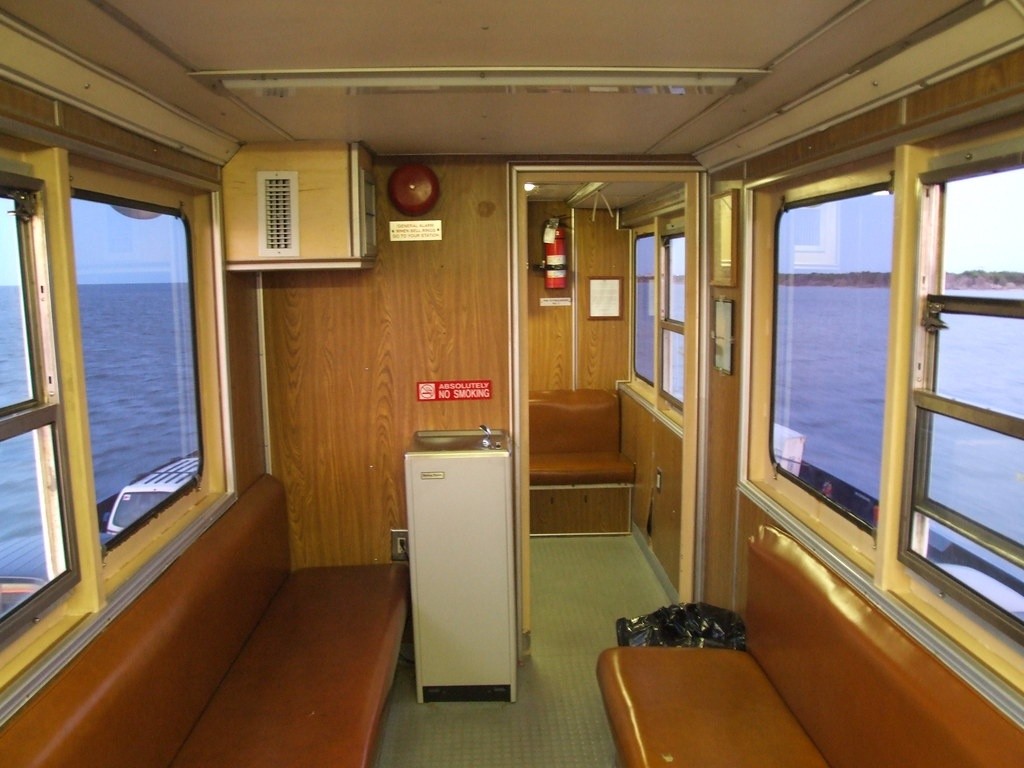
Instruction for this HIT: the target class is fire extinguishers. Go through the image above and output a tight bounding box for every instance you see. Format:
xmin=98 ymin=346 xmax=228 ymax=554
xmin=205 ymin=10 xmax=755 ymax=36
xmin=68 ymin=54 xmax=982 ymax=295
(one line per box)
xmin=543 ymin=215 xmax=571 ymax=289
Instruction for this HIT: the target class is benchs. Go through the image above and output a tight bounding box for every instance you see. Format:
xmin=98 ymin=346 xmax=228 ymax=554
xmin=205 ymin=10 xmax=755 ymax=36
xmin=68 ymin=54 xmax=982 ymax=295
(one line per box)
xmin=0 ymin=473 xmax=410 ymax=768
xmin=596 ymin=525 xmax=1024 ymax=768
xmin=529 ymin=389 xmax=636 ymax=537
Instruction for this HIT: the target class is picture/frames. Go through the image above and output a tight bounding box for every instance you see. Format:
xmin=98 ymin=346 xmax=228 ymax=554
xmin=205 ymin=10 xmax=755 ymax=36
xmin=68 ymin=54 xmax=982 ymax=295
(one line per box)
xmin=585 ymin=275 xmax=624 ymax=320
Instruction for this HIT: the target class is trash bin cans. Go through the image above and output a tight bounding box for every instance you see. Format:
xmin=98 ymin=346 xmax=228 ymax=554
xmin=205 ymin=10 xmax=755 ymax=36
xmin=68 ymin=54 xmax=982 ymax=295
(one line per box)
xmin=615 ymin=602 xmax=747 ymax=650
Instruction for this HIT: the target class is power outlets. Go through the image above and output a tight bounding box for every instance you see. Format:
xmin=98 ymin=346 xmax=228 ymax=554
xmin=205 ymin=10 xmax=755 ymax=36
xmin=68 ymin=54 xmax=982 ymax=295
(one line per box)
xmin=390 ymin=530 xmax=409 ymax=561
